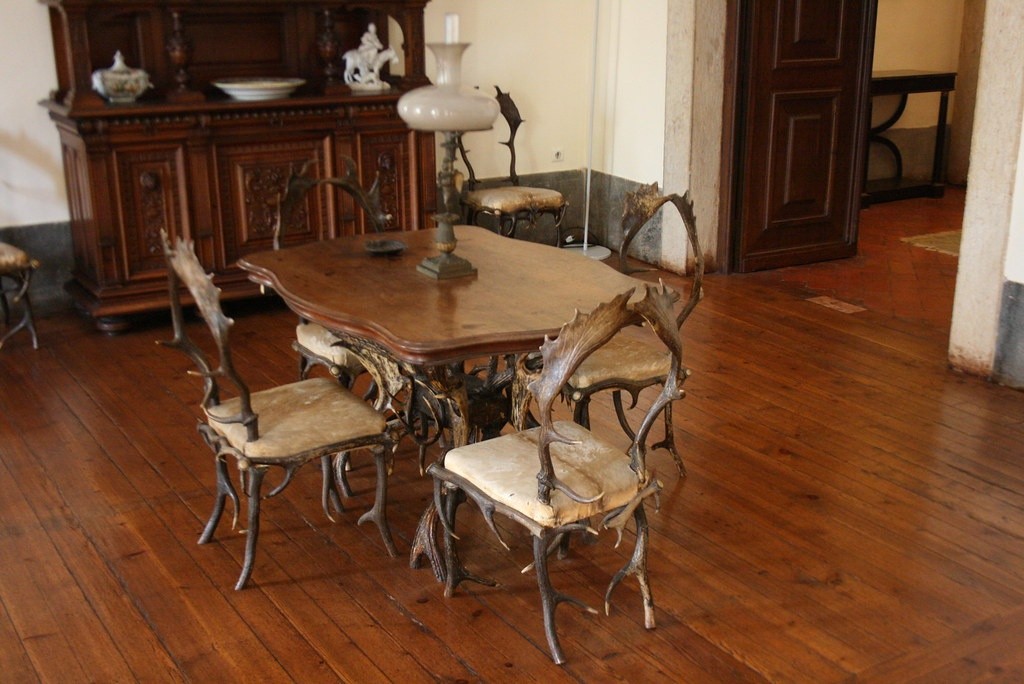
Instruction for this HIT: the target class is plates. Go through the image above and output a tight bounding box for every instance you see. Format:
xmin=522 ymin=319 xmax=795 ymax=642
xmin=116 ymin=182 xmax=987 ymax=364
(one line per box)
xmin=364 ymin=239 xmax=407 ymax=256
xmin=209 ymin=77 xmax=307 ymax=101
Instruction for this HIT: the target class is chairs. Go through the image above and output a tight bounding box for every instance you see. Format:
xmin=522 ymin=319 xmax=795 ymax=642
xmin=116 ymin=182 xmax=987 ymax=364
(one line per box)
xmin=449 ymin=85 xmax=569 ymax=248
xmin=271 ymin=153 xmax=394 ymax=388
xmin=422 ymin=276 xmax=691 ymax=667
xmin=507 ymin=180 xmax=704 ymax=477
xmin=148 ymin=229 xmax=401 ymax=591
xmin=0 ymin=239 xmax=40 ymax=353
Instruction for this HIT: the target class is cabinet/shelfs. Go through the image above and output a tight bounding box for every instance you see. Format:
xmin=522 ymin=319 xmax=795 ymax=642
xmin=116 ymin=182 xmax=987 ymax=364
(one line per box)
xmin=862 ymin=69 xmax=957 ymax=207
xmin=37 ymin=1 xmax=438 ymax=337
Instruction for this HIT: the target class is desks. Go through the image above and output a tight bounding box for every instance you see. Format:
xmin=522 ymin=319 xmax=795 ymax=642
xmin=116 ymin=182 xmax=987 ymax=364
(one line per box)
xmin=238 ymin=224 xmax=680 ymax=584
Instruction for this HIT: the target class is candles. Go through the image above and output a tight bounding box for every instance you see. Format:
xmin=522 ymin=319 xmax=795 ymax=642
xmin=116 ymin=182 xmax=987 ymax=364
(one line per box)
xmin=446 ymin=15 xmax=460 ymax=43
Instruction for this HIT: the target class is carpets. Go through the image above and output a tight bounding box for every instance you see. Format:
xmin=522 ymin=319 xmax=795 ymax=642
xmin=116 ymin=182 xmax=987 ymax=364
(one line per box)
xmin=900 ymin=229 xmax=961 ymax=256
